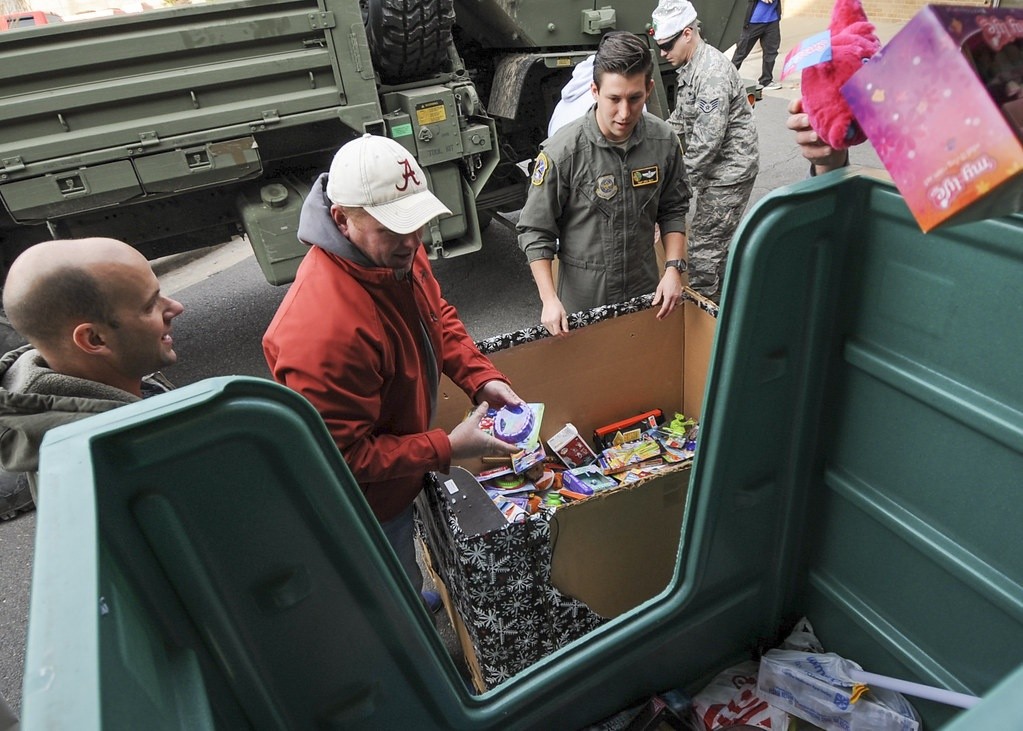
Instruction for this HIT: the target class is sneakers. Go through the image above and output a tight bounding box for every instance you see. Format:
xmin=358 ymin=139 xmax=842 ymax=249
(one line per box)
xmin=763 ymin=81 xmax=782 ymax=90
xmin=421 ymin=592 xmax=442 ymax=614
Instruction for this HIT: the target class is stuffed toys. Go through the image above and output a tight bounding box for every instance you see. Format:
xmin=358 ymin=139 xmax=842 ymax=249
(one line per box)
xmin=801 ymin=2 xmax=883 ymax=151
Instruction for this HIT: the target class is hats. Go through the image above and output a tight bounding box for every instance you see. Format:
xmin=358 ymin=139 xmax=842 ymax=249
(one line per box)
xmin=326 ymin=133 xmax=452 ymax=234
xmin=651 ymin=0 xmax=697 ymax=45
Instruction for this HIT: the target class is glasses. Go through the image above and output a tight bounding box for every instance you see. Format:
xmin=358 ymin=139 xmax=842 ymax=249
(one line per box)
xmin=656 ymin=27 xmax=693 ymax=52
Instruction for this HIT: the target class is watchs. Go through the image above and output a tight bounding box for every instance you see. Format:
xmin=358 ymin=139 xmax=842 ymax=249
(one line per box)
xmin=664 ymin=257 xmax=689 ymax=275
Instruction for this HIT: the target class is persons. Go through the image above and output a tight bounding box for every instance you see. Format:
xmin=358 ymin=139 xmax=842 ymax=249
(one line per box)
xmin=547 ymin=53 xmax=598 ymax=140
xmin=732 ymin=0 xmax=782 ymax=90
xmin=261 ymin=134 xmax=525 ymax=618
xmin=515 ymin=31 xmax=694 ymax=336
xmin=0 ymin=235 xmax=188 ymax=529
xmin=787 ymin=94 xmax=850 ymax=177
xmin=652 ymin=0 xmax=761 ymax=297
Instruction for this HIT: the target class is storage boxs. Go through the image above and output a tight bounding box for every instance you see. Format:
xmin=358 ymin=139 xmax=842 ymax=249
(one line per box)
xmin=19 ymin=161 xmax=1023 ymax=731
xmin=839 ymin=3 xmax=1022 ymax=236
xmin=411 ymin=285 xmax=723 ymax=696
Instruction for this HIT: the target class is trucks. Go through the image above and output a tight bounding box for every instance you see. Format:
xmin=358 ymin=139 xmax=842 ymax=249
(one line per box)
xmin=0 ymin=0 xmax=757 ymax=287
